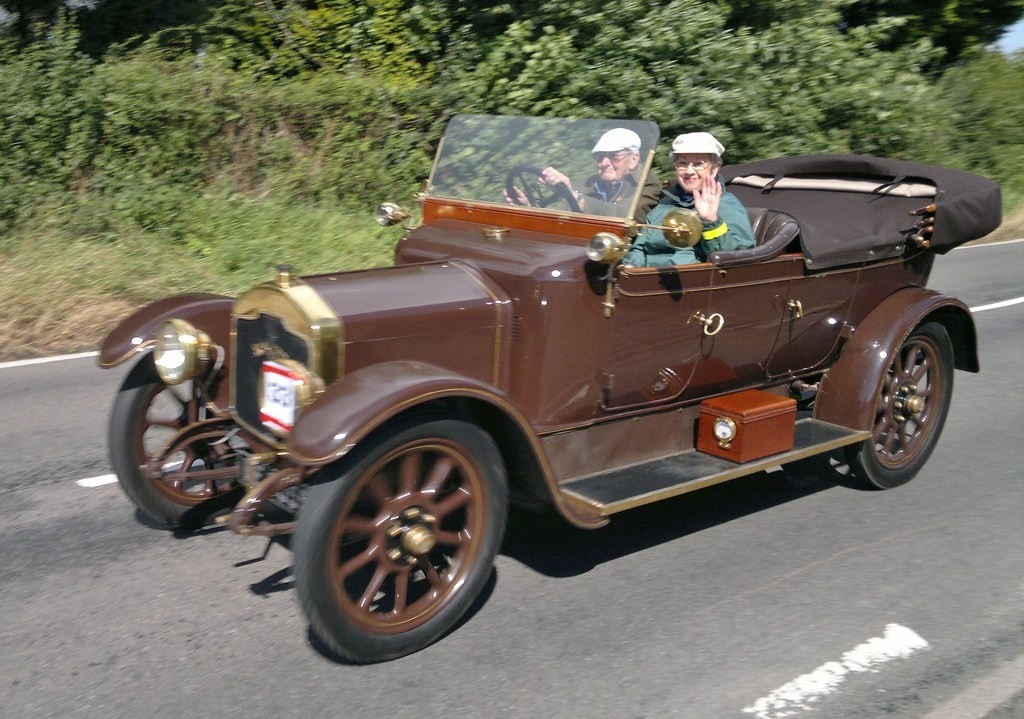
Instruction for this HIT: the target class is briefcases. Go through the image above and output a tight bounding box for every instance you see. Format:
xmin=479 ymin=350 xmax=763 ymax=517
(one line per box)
xmin=696 ymin=388 xmax=797 ymax=466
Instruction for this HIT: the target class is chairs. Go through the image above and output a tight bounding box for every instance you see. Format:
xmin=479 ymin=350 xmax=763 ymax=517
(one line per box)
xmin=710 ymin=208 xmax=800 ymax=266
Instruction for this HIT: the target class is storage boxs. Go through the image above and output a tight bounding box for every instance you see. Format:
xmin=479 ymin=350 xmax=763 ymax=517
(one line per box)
xmin=696 ymin=388 xmax=798 ymax=463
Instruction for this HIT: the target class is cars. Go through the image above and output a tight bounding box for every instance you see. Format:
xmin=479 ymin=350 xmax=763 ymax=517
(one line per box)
xmin=96 ymin=112 xmax=1001 ymax=672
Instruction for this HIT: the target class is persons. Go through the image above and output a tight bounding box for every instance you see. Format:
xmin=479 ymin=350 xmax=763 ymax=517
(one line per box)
xmin=619 ymin=130 xmax=758 ymax=270
xmin=503 ymin=127 xmax=663 ymax=224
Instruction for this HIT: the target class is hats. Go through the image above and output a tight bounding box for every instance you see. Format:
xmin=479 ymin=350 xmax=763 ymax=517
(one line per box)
xmin=672 ymin=132 xmax=726 ymax=157
xmin=591 ymin=128 xmax=642 ymax=157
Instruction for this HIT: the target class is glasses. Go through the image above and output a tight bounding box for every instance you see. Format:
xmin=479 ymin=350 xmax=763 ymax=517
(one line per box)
xmin=594 ymin=152 xmax=634 ymax=161
xmin=673 ymin=159 xmax=715 ymax=171
